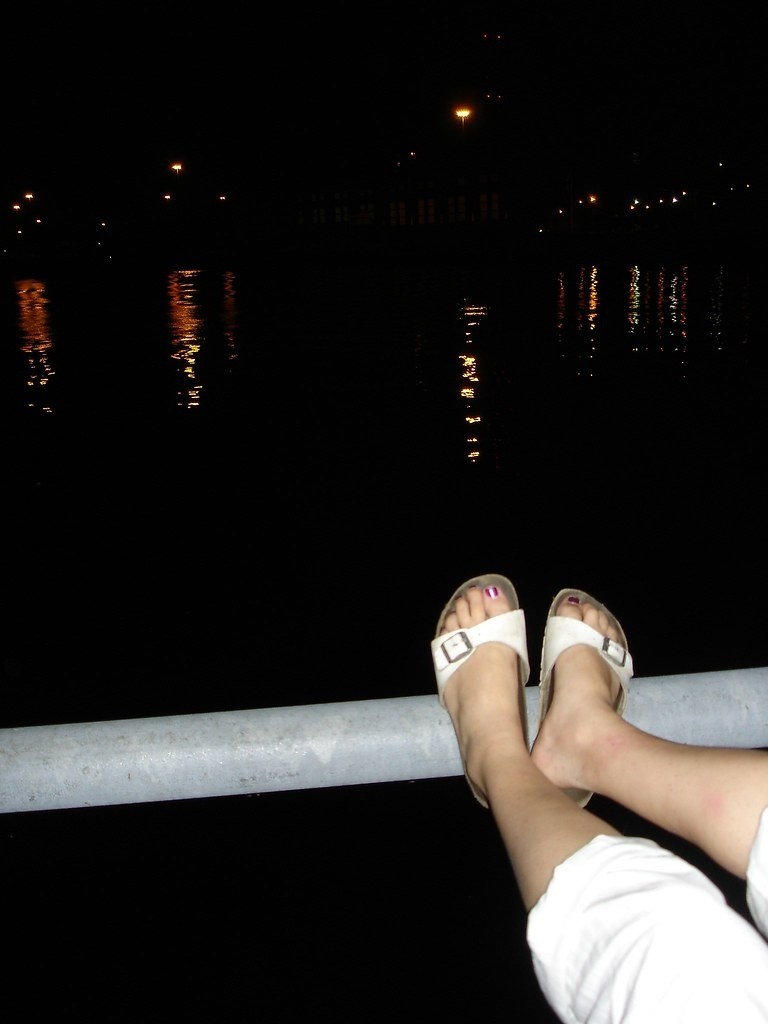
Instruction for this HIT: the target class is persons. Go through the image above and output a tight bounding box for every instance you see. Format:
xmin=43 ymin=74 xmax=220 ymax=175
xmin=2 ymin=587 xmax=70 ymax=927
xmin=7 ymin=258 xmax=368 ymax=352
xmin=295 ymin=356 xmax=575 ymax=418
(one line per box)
xmin=430 ymin=574 xmax=768 ymax=1024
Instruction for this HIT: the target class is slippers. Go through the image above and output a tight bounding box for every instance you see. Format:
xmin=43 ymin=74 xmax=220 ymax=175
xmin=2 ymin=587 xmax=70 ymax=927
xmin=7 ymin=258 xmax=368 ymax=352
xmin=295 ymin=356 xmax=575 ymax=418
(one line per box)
xmin=430 ymin=574 xmax=531 ymax=810
xmin=539 ymin=588 xmax=634 ymax=809
